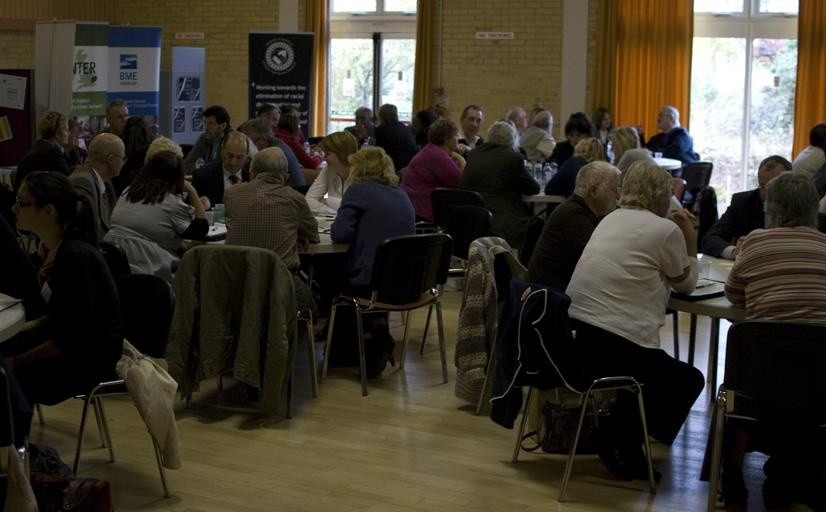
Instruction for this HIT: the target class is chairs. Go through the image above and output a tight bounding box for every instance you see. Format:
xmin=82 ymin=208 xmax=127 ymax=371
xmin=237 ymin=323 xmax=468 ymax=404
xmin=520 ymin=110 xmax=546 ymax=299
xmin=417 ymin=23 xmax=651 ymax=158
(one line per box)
xmin=445 ymin=205 xmax=494 ymax=258
xmin=468 ymin=237 xmax=516 ymax=415
xmin=67 ymin=271 xmax=176 ymax=498
xmin=429 ymin=186 xmax=484 ymax=227
xmin=99 ymin=242 xmax=131 ymax=274
xmin=321 ymin=233 xmax=452 ymax=396
xmin=177 ymin=244 xmax=319 ymax=419
xmin=705 ymin=320 xmax=826 ymax=511
xmin=511 ymin=277 xmax=656 ymax=503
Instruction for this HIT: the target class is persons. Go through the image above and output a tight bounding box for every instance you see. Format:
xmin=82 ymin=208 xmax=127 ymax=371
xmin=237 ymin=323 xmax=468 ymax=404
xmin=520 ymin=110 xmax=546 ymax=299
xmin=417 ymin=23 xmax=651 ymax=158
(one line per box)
xmin=374 ymin=104 xmax=419 ymax=171
xmin=326 ymin=147 xmax=415 ymax=378
xmin=304 ymin=132 xmax=358 ymax=215
xmin=811 ymin=161 xmax=826 ymax=232
xmin=459 ymin=121 xmax=545 ymax=244
xmin=544 ymin=137 xmax=605 ymax=221
xmin=13 ymin=110 xmax=77 ymax=191
xmin=65 ymin=119 xmax=87 ymax=163
xmin=186 ymin=134 xmax=253 ymax=212
xmin=272 ymin=113 xmax=322 ymax=169
xmin=411 ymin=111 xmax=434 ymax=146
xmin=111 ymin=116 xmax=151 ymax=198
xmin=518 ymin=111 xmax=557 ymax=163
xmin=565 ymin=160 xmax=705 ymax=482
xmin=256 ymin=104 xmax=280 ymax=130
xmin=505 ymin=106 xmax=528 ymax=134
xmin=68 ymin=132 xmax=128 ymax=239
xmin=545 ymin=111 xmax=611 ymax=170
xmin=222 ymin=147 xmax=319 ymax=398
xmin=1 ymin=170 xmax=121 ymax=448
xmin=241 ymin=120 xmax=306 ymax=186
xmin=402 ymin=116 xmax=467 ymax=228
xmin=499 ymin=119 xmax=527 ymax=160
xmin=607 ymin=126 xmax=685 ymax=214
xmin=102 ymin=152 xmax=209 ymax=305
xmin=88 ymin=99 xmax=128 ymax=147
xmin=182 ymin=106 xmax=259 ymax=179
xmin=144 ymin=136 xmax=210 ymax=210
xmin=701 ymin=155 xmax=793 ymax=260
xmin=592 ymin=108 xmax=613 ymax=145
xmin=455 ymin=105 xmax=484 ymax=160
xmin=699 ymin=170 xmax=826 ymax=511
xmin=281 ymin=104 xmax=310 ymax=154
xmin=790 ymin=125 xmax=826 ymax=176
xmin=636 ymin=105 xmax=698 ymax=177
xmin=528 ymin=161 xmax=621 ymax=292
xmin=344 ymin=108 xmax=376 ymax=148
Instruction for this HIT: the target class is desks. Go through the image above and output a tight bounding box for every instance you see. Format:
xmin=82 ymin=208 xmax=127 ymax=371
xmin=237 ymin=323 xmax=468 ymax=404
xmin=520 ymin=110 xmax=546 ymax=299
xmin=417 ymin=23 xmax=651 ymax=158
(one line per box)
xmin=664 ymin=251 xmax=751 ymax=413
xmin=521 ymin=181 xmax=565 ymax=217
xmin=203 ymin=206 xmax=348 ymax=256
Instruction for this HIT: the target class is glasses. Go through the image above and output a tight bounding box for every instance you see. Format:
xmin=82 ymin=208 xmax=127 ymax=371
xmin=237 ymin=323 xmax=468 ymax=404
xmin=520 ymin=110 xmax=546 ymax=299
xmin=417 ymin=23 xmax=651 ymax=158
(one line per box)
xmin=116 ymin=156 xmax=128 ymax=162
xmin=15 ymin=196 xmax=31 ymax=208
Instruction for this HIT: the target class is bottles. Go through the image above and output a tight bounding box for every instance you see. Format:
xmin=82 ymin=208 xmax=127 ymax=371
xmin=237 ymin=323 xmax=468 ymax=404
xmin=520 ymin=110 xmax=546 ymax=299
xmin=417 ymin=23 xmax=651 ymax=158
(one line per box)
xmin=534 ymin=160 xmax=542 ymax=180
xmin=303 ymin=141 xmax=310 ymax=156
xmin=542 ymin=163 xmax=552 ymax=184
xmin=551 ymin=159 xmax=557 ymax=174
xmin=526 ymin=160 xmax=532 ymax=176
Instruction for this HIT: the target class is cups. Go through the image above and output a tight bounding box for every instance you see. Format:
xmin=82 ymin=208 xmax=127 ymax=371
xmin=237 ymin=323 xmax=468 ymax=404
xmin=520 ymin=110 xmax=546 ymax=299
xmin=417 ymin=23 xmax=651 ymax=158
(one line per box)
xmin=698 ymin=259 xmax=712 ymax=283
xmin=655 ymin=152 xmax=662 ymax=160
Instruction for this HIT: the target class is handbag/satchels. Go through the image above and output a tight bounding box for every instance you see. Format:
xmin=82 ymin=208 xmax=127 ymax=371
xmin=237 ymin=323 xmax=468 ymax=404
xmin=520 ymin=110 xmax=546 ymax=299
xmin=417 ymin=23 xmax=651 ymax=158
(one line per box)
xmin=520 ymin=403 xmax=586 ymax=457
xmin=56 ymin=476 xmax=112 ymax=512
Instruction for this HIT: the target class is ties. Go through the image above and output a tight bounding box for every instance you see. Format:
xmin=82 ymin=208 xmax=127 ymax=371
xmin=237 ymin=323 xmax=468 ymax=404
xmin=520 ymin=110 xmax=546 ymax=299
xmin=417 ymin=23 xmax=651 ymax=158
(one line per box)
xmin=228 ymin=174 xmax=240 ymax=185
